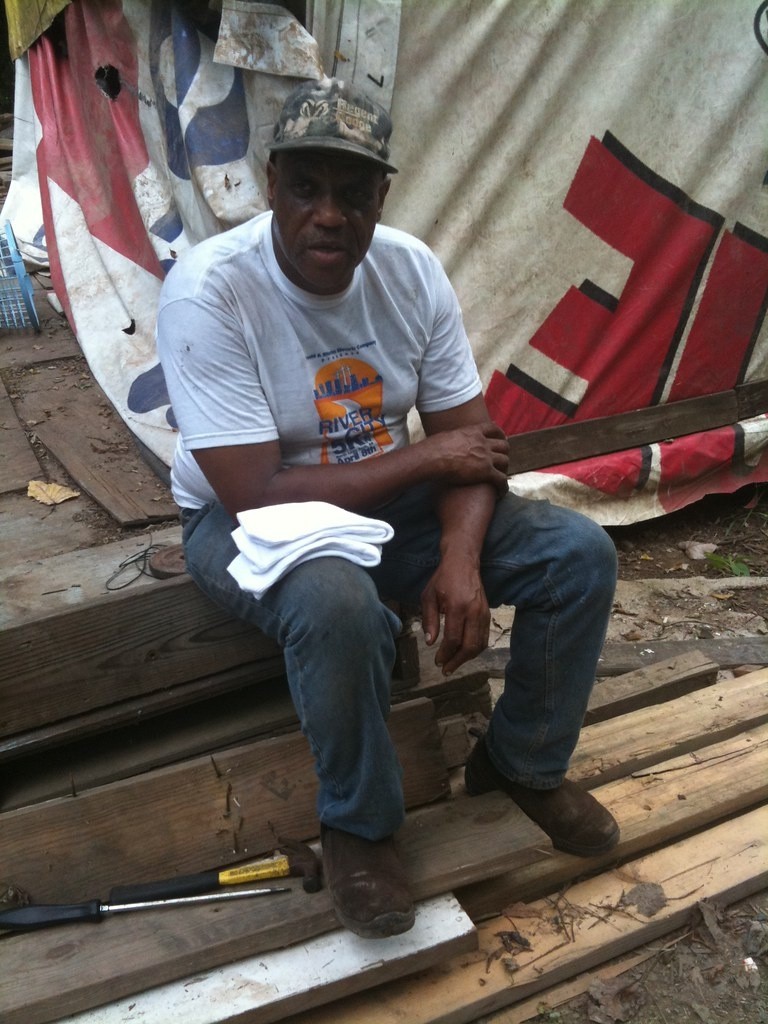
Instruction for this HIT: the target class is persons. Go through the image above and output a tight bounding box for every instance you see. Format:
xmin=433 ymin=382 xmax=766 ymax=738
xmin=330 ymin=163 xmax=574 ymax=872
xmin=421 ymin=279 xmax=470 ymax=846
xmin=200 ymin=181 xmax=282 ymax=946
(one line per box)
xmin=157 ymin=79 xmax=618 ymax=939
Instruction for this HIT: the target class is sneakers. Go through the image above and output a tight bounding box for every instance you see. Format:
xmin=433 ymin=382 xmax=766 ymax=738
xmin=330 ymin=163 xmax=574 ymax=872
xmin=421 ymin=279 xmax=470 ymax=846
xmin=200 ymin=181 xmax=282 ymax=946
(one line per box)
xmin=318 ymin=815 xmax=414 ymax=939
xmin=463 ymin=739 xmax=620 ymax=855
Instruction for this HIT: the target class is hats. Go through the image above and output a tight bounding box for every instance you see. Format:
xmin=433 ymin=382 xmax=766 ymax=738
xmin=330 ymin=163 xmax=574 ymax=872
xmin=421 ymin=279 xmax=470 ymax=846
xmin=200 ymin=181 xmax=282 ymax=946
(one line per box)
xmin=268 ymin=77 xmax=398 ymax=175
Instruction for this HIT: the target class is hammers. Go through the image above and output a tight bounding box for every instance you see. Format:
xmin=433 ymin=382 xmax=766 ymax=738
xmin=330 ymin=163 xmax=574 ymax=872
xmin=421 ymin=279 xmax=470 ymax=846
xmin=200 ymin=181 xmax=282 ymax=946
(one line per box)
xmin=107 ymin=832 xmax=325 ymax=896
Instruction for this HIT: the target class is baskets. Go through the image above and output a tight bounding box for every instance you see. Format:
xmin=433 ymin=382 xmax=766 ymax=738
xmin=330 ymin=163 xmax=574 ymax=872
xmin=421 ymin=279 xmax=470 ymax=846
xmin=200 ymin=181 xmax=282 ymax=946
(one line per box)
xmin=0 ymin=218 xmax=41 ymax=331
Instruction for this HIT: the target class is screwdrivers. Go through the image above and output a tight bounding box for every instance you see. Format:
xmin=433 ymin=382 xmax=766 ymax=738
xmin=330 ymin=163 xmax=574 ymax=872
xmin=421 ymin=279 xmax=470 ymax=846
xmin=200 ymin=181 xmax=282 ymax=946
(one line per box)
xmin=0 ymin=885 xmax=294 ymax=934
